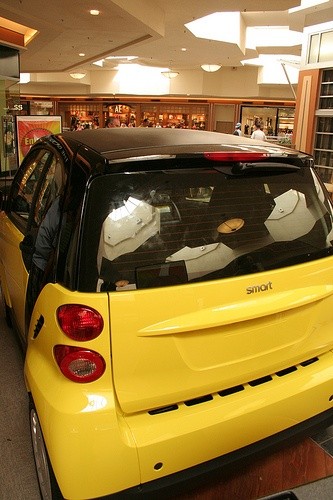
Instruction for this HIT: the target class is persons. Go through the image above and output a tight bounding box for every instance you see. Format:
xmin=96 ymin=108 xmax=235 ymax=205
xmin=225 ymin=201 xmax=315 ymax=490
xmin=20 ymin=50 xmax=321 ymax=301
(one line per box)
xmin=250 ymin=123 xmax=267 ymax=142
xmin=233 ymin=123 xmax=242 ymax=136
xmin=71 ymin=111 xmax=206 ymax=131
xmin=24 ymin=179 xmax=74 ymax=338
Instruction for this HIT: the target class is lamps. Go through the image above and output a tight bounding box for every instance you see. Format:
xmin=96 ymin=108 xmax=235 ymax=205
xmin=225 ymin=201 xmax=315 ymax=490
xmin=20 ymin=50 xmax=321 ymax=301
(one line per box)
xmin=161 ymin=60 xmax=180 ymax=79
xmin=199 ymin=63 xmax=223 ymax=73
xmin=69 ymin=72 xmax=87 ymax=79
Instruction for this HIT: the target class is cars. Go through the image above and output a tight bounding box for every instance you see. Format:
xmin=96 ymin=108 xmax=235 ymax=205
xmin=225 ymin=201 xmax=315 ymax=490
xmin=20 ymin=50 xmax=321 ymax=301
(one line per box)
xmin=0 ymin=126 xmax=333 ymax=500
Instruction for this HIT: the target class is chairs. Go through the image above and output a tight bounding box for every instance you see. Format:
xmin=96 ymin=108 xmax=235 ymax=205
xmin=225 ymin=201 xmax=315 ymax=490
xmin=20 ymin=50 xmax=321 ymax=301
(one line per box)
xmin=185 ymin=181 xmax=263 ymax=250
